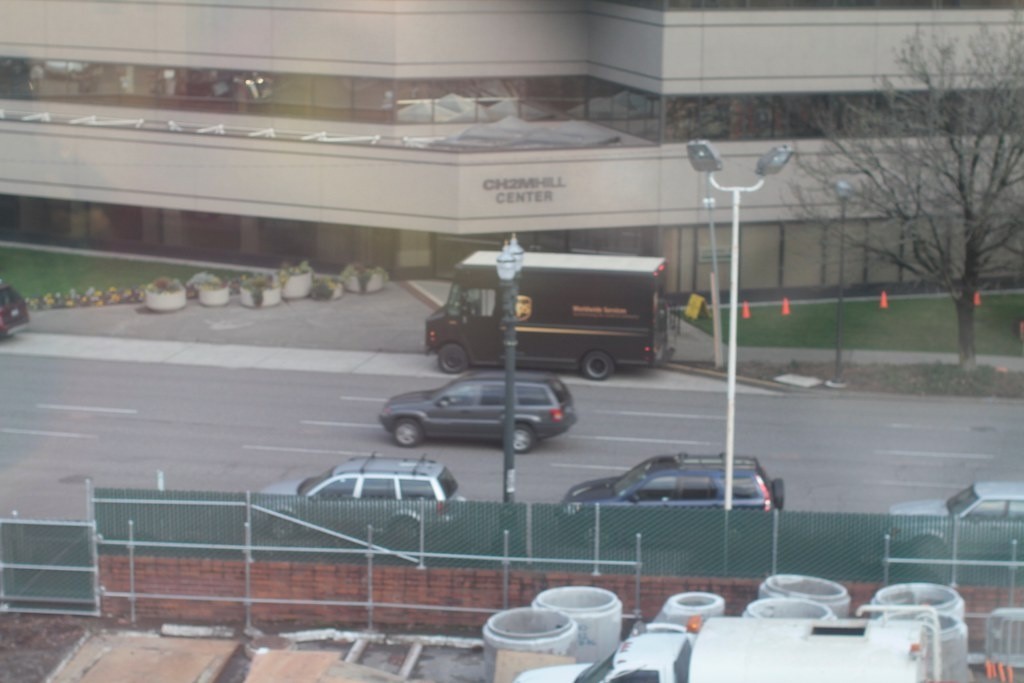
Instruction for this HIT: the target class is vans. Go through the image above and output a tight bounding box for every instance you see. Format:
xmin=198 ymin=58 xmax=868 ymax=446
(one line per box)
xmin=425 ymin=249 xmax=669 ymax=380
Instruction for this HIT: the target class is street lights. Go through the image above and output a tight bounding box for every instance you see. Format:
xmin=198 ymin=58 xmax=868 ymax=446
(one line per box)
xmin=687 ymin=138 xmax=794 ymax=576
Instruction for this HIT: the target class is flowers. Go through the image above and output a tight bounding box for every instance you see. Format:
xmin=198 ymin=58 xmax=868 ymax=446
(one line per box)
xmin=142 ymin=263 xmax=385 ymax=304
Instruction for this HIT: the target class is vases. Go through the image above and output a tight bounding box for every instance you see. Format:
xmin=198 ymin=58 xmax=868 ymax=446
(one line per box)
xmin=312 ymin=285 xmax=342 ymax=300
xmin=239 ymin=285 xmax=281 ymax=308
xmin=272 ymin=272 xmax=313 ymax=299
xmin=144 ymin=287 xmax=186 ymax=311
xmin=343 ymin=273 xmax=384 ymax=293
xmin=198 ymin=285 xmax=229 ymax=306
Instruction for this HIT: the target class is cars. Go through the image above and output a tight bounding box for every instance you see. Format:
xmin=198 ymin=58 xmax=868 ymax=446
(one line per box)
xmin=1 ymin=285 xmax=29 ymax=340
xmin=882 ymin=482 xmax=1024 ymax=572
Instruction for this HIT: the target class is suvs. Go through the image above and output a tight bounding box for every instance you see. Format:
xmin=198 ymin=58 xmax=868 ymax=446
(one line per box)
xmin=379 ymin=371 xmax=576 ymax=456
xmin=561 ymin=452 xmax=785 ymax=549
xmin=254 ymin=453 xmax=467 ymax=548
